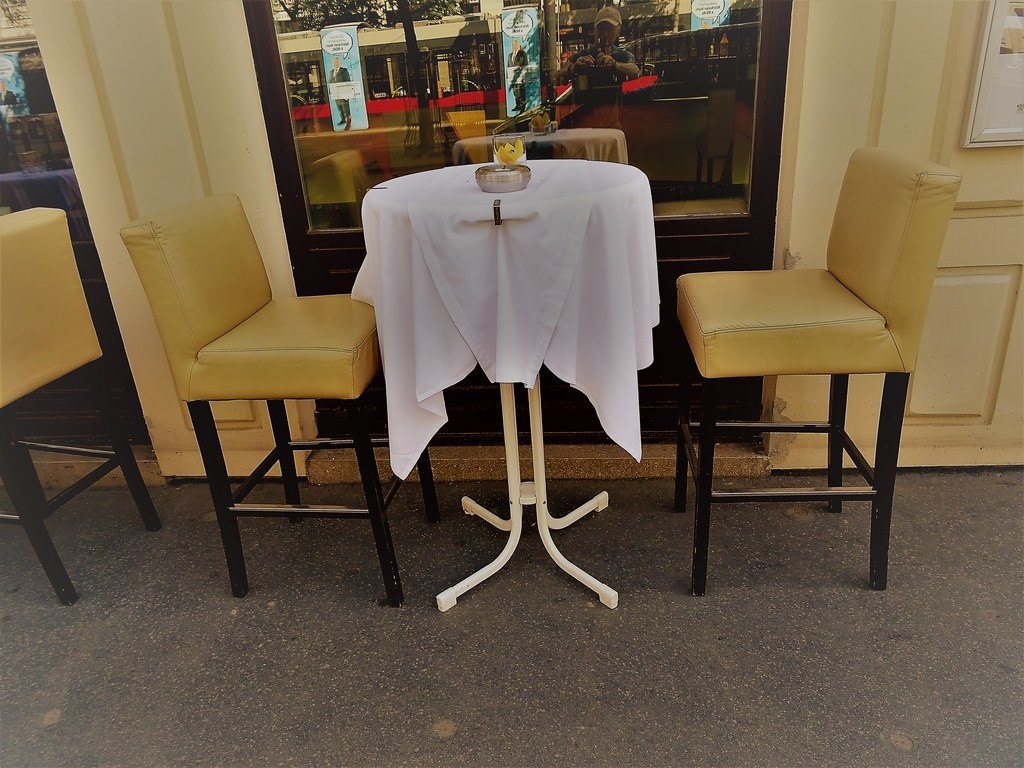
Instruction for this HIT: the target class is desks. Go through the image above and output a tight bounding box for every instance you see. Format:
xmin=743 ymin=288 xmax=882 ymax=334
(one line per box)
xmin=351 ymin=158 xmax=662 ymax=614
xmin=455 ymin=128 xmax=630 ymax=168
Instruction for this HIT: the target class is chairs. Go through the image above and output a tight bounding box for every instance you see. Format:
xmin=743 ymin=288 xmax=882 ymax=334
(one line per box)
xmin=0 ymin=191 xmax=443 ymax=604
xmin=670 ymin=144 xmax=963 ymax=597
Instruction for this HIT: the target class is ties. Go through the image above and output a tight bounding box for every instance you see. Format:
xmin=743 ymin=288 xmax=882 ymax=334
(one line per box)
xmin=335 ymin=69 xmax=338 ymax=82
xmin=513 ymin=53 xmax=516 ymax=63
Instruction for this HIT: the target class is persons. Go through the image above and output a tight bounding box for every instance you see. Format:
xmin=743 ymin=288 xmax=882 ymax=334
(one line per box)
xmin=0 ymin=81 xmax=18 ymax=104
xmin=328 ymin=56 xmax=352 ymax=131
xmin=507 ymin=39 xmax=528 ymax=115
xmin=553 ymin=7 xmax=640 ymax=84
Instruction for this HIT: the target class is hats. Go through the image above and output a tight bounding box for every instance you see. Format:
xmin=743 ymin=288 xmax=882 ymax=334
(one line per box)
xmin=595 ymin=7 xmax=622 ymax=27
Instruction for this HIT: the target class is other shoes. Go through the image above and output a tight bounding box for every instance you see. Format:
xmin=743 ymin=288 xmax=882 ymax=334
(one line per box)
xmin=518 ymin=106 xmax=525 ymax=114
xmin=345 ymin=122 xmax=351 ymax=130
xmin=338 ymin=119 xmax=346 ymax=125
xmin=512 ymin=105 xmax=521 ymax=111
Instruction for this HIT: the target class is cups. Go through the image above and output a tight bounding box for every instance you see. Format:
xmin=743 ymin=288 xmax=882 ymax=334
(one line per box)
xmin=530 ymin=109 xmax=550 ymax=136
xmin=492 ymin=132 xmax=526 ymax=166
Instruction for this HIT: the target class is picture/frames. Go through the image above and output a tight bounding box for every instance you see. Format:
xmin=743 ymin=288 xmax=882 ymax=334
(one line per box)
xmin=959 ymin=0 xmax=1024 ymax=150
